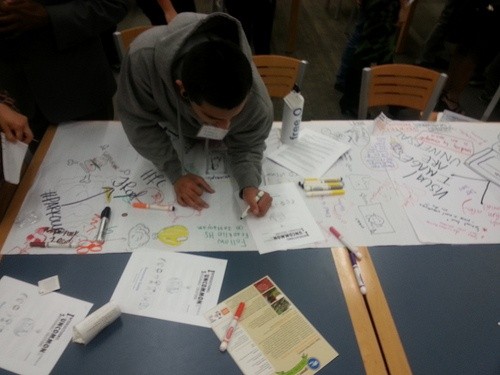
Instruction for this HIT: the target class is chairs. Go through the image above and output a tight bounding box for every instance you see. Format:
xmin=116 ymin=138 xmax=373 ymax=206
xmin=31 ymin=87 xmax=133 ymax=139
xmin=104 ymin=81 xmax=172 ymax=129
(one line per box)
xmin=250 ymin=53 xmax=450 ymax=123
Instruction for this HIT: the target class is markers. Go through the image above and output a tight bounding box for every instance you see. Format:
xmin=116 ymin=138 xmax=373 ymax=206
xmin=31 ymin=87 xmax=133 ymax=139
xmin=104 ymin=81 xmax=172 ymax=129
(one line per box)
xmin=130 ymin=201 xmax=174 ymax=213
xmin=297 ymin=177 xmax=346 ymax=198
xmin=219 ymin=302 xmax=245 ymax=352
xmin=327 ymin=225 xmax=365 ymax=259
xmin=239 ymin=191 xmax=264 ymax=219
xmin=96 ymin=206 xmax=111 ymax=243
xmin=346 ymin=251 xmax=368 ymax=296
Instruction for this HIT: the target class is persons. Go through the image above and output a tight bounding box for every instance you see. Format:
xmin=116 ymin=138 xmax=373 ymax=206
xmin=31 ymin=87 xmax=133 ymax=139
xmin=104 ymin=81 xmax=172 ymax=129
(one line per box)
xmin=333 ymin=0 xmax=415 ymax=119
xmin=0 ymin=0 xmax=131 ymax=153
xmin=113 ymin=13 xmax=273 ymax=218
xmin=399 ymin=1 xmax=500 ymax=109
xmin=89 ymin=0 xmax=279 ymax=73
xmin=0 ymin=88 xmax=33 ymax=159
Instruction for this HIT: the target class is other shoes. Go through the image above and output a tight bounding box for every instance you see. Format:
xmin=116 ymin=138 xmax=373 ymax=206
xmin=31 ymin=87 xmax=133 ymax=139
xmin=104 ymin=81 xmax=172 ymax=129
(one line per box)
xmin=340 ymin=94 xmax=359 ymax=115
xmin=438 ymin=90 xmax=466 ymax=114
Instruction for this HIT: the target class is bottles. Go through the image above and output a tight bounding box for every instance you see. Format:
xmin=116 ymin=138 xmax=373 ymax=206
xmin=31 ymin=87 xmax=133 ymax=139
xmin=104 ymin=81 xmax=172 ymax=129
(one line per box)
xmin=282 ymin=83 xmax=303 ymax=143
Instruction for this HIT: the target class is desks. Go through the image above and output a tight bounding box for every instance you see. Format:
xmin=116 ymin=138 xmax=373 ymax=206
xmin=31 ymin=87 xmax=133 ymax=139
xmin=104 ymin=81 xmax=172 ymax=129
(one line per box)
xmin=0 ymin=113 xmax=500 ymax=374
